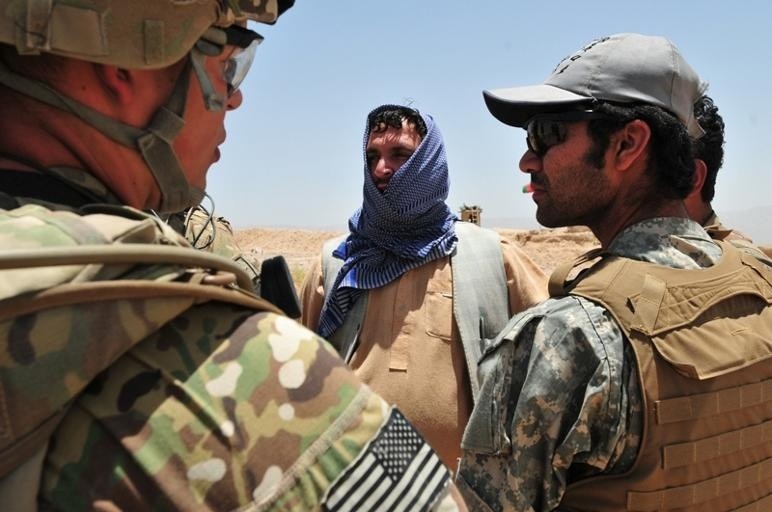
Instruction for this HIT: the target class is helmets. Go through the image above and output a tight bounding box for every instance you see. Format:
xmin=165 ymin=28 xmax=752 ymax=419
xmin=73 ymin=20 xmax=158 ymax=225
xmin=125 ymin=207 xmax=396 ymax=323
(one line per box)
xmin=0 ymin=0 xmax=296 ymax=71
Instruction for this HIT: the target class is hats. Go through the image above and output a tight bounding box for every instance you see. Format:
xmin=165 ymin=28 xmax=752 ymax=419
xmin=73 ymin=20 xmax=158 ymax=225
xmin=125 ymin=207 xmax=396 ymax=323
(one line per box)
xmin=483 ymin=32 xmax=707 ymax=139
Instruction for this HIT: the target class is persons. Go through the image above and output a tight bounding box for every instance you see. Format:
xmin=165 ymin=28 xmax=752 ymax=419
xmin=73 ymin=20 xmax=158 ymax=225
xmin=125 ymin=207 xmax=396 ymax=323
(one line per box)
xmin=182 ymin=209 xmax=262 ymax=296
xmin=681 ymin=93 xmax=772 ymax=266
xmin=296 ymin=104 xmax=550 ymax=479
xmin=482 ymin=35 xmax=772 ymax=511
xmin=0 ymin=1 xmax=468 ymax=512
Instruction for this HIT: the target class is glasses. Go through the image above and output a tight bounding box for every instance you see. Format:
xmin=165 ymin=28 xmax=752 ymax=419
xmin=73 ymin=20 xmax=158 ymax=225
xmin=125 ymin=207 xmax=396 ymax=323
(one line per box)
xmin=202 ymin=25 xmax=264 ymax=94
xmin=526 ymin=112 xmax=596 ymax=155
